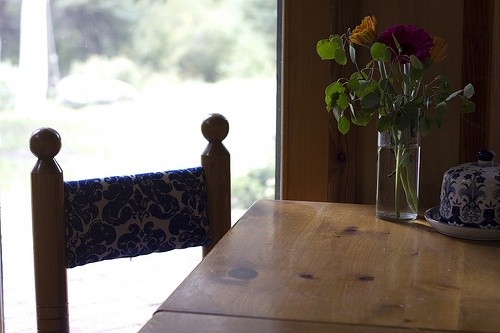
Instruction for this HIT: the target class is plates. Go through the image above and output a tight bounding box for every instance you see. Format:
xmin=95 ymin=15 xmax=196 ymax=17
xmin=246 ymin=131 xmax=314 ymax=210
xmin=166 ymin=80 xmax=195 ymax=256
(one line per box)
xmin=424 ymin=207 xmax=500 ymax=241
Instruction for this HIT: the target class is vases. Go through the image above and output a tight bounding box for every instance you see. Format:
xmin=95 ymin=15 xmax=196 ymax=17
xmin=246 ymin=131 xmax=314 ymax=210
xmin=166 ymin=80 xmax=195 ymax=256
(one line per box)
xmin=374 ymin=121 xmax=423 ymax=225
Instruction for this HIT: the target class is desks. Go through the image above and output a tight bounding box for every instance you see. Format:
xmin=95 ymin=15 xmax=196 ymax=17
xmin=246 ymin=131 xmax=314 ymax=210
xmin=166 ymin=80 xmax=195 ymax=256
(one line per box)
xmin=137 ymin=199 xmax=500 ymax=332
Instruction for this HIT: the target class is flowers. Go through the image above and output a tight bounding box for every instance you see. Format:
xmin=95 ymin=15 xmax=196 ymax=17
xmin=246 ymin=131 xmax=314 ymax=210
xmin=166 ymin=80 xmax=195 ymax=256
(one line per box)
xmin=314 ymin=15 xmax=477 ymax=218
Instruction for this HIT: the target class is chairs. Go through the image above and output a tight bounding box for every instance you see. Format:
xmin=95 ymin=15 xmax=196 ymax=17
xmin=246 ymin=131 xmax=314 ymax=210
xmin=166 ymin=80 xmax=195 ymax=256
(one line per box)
xmin=27 ymin=111 xmax=233 ymax=333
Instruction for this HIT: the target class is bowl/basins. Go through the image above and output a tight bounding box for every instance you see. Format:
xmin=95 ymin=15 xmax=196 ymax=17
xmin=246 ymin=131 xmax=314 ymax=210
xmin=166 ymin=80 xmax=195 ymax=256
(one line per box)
xmin=438 ymin=160 xmax=499 ymax=230
